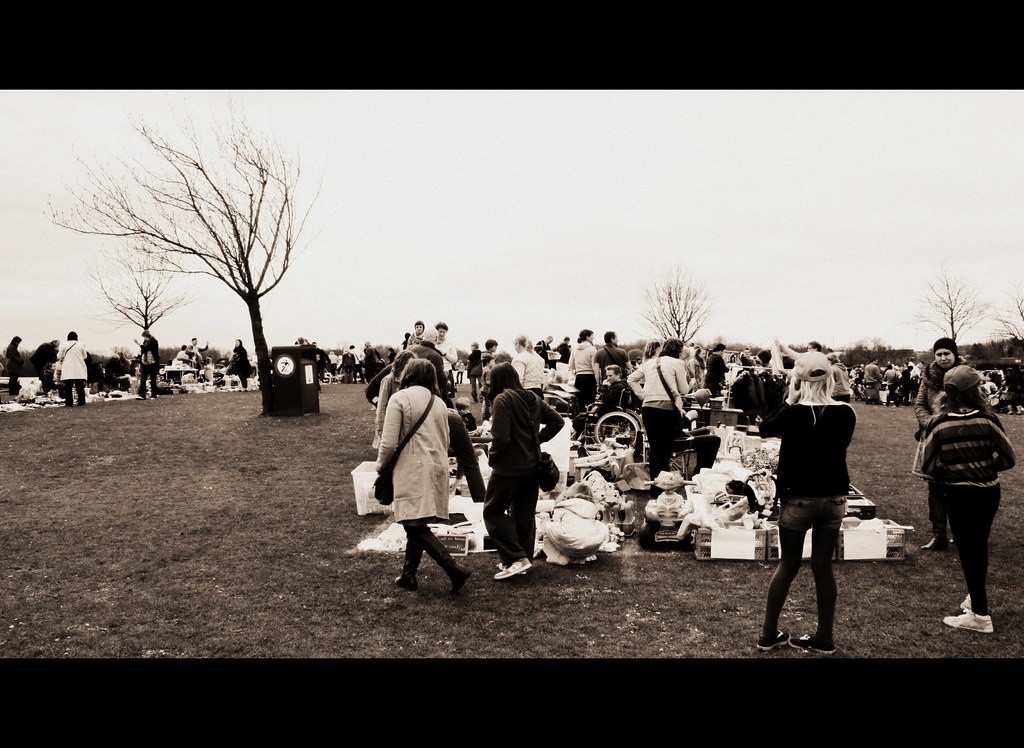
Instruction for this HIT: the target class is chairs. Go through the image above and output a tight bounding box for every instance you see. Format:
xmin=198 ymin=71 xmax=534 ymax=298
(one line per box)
xmin=643 ymin=430 xmax=694 ymax=480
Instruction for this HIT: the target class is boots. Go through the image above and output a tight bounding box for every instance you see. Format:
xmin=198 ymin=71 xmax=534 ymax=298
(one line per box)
xmin=394 ymin=574 xmax=417 ymax=590
xmin=448 ymin=563 xmax=472 ymax=592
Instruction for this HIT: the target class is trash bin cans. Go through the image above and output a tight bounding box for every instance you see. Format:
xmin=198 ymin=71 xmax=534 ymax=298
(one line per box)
xmin=272 ymin=344 xmax=320 ymax=416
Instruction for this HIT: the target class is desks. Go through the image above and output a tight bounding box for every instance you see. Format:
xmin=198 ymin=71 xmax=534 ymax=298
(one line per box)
xmin=165 ymin=369 xmax=198 ymax=386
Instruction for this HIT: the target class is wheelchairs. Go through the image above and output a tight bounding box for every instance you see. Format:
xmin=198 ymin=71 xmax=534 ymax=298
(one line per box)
xmin=572 ymin=388 xmax=642 ymax=450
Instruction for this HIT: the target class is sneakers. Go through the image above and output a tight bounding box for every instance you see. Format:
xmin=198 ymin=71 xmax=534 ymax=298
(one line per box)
xmin=943 ymin=611 xmax=993 ymax=633
xmin=494 ymin=558 xmax=531 ymax=579
xmin=960 ymin=593 xmax=972 ymax=612
xmin=497 ymin=563 xmax=527 ymax=574
xmin=757 ymin=630 xmax=791 ymax=649
xmin=788 ymin=633 xmax=835 ymax=653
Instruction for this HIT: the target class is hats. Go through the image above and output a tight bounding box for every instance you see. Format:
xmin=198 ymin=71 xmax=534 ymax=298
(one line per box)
xmin=943 ymin=365 xmax=991 ymax=391
xmin=794 ymin=352 xmax=832 ymax=381
xmin=933 ymin=338 xmax=957 ymax=357
xmin=141 ymin=331 xmax=151 ymax=336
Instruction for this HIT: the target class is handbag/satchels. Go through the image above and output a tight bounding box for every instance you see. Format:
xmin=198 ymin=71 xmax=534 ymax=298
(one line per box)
xmin=538 ymin=452 xmax=558 ymax=491
xmin=371 ymin=460 xmax=397 ymax=505
xmin=57 ymin=359 xmax=63 ymax=370
xmin=359 ymin=361 xmax=365 ymax=368
xmin=142 ymin=351 xmax=154 ymax=365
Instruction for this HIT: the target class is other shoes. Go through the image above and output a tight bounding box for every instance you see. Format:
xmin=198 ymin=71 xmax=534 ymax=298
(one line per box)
xmin=353 ymin=381 xmax=357 ymax=384
xmin=474 ymin=399 xmax=478 ymax=403
xmin=921 ymin=536 xmax=949 ymax=551
xmin=136 ymin=396 xmax=144 ymax=400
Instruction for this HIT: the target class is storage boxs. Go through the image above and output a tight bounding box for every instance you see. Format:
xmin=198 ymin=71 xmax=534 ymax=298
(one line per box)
xmin=350 ymin=462 xmax=394 ymax=515
xmin=695 ymin=484 xmax=905 ymax=560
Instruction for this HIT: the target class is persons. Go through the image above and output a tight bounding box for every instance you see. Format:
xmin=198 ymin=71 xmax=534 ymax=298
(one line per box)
xmin=756 ymin=350 xmax=858 ymax=655
xmin=922 ymin=367 xmax=1016 ymax=634
xmin=3 ymin=321 xmax=1023 ymax=594
